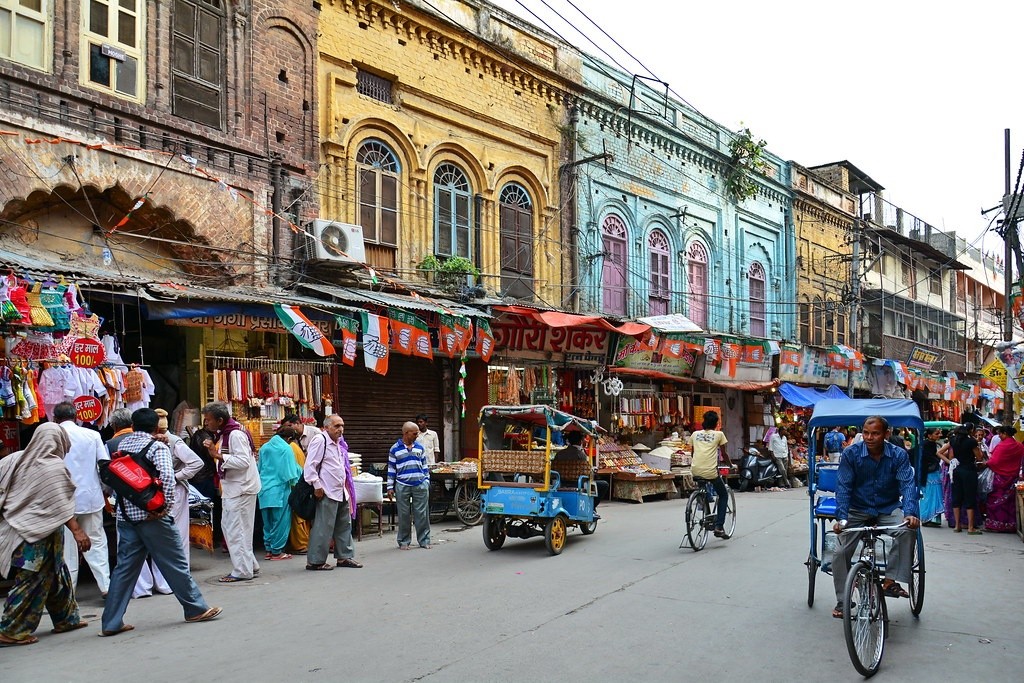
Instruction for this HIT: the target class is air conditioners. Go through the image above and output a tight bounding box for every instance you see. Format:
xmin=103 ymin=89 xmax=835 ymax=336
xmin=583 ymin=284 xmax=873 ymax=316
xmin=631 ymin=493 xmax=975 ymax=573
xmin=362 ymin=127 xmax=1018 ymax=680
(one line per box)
xmin=303 ymin=218 xmax=366 ymax=267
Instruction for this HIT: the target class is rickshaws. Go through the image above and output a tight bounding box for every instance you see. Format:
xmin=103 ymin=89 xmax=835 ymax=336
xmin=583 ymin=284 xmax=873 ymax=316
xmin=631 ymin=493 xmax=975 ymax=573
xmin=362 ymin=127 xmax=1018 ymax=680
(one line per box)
xmin=428 ymin=477 xmax=485 ymax=525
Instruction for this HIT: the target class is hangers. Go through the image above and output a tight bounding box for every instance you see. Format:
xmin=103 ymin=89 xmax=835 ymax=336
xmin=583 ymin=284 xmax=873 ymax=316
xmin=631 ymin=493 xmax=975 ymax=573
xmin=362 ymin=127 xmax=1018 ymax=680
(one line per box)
xmin=210 ymin=356 xmax=335 ymax=379
xmin=612 ymin=412 xmax=690 ymax=421
xmin=0 ymin=267 xmax=80 ymax=297
xmin=489 ymin=355 xmax=597 ymax=380
xmin=617 ymin=389 xmax=692 ymax=402
xmin=0 ymin=354 xmax=149 ymax=381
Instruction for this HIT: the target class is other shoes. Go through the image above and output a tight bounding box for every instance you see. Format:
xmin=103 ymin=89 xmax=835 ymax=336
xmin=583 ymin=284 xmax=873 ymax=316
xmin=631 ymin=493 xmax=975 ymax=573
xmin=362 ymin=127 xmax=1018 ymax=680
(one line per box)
xmin=925 ymin=523 xmax=940 ymax=527
xmin=697 ymin=503 xmax=704 ymax=511
xmin=712 ymin=530 xmax=729 ymax=539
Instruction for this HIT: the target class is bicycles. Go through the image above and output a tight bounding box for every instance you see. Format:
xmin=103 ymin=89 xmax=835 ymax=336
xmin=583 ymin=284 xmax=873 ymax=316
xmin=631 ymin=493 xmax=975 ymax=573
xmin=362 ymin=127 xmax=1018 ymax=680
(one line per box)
xmin=679 ymin=467 xmax=737 ymax=551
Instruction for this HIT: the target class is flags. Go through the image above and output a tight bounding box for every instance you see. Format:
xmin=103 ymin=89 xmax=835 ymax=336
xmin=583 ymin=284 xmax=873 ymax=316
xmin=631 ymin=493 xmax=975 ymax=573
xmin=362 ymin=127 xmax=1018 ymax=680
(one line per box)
xmin=639 ymin=328 xmax=1003 ymax=417
xmin=273 ymin=301 xmax=496 ymax=376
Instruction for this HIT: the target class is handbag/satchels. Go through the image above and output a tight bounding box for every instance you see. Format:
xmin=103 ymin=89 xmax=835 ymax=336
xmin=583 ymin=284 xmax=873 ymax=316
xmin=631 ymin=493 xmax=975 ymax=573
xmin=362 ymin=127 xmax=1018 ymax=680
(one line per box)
xmin=97 ymin=440 xmax=167 ymax=513
xmin=287 ymin=471 xmax=316 ymax=521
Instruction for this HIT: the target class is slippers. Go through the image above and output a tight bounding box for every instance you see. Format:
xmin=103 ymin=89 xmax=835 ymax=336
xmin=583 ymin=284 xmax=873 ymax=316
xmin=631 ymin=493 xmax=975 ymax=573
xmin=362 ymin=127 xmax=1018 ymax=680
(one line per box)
xmin=306 ymin=562 xmax=334 ymax=570
xmin=51 ymin=622 xmax=88 ymax=633
xmin=97 ymin=624 xmax=135 ymax=637
xmin=337 ymin=558 xmax=363 ymax=568
xmin=0 ymin=636 xmax=39 ymax=647
xmin=883 ymin=582 xmax=910 ymax=598
xmin=832 ymin=607 xmax=843 ymax=618
xmin=967 ymin=530 xmax=982 ymax=534
xmin=270 ymin=553 xmax=295 ymax=560
xmin=952 ymin=528 xmax=963 ymax=532
xmin=185 ymin=607 xmax=223 ymax=622
xmin=218 ymin=574 xmax=242 ymax=581
xmin=264 ymin=554 xmax=272 ymax=560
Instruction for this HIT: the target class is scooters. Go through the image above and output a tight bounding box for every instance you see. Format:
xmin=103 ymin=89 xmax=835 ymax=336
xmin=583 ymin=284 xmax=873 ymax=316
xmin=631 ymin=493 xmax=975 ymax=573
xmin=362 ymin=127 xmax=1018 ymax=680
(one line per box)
xmin=737 ymin=446 xmax=782 ymax=492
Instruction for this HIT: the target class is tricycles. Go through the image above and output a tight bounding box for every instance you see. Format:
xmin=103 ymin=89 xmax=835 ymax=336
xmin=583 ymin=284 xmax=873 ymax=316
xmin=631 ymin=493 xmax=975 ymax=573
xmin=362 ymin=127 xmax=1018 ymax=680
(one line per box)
xmin=810 ymin=399 xmax=927 ymax=675
xmin=480 ymin=406 xmax=600 ymax=556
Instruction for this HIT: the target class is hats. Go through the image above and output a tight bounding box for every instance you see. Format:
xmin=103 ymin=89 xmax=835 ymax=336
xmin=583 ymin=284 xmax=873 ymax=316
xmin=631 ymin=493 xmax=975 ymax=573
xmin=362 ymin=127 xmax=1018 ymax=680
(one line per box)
xmin=154 ymin=408 xmax=169 ymax=429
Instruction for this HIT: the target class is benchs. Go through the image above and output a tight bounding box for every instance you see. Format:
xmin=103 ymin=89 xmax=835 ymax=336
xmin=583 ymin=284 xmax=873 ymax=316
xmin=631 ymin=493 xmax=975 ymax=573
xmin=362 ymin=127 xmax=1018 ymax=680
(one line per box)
xmin=815 ymin=462 xmax=840 ymax=520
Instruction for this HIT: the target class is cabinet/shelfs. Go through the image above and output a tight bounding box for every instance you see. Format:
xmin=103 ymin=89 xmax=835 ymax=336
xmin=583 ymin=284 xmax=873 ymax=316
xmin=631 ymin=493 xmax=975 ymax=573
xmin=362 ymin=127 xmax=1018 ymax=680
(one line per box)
xmin=351 ymin=502 xmax=383 ymax=542
xmin=612 ymin=479 xmax=678 ymax=504
xmin=596 ymin=435 xmax=648 ymax=502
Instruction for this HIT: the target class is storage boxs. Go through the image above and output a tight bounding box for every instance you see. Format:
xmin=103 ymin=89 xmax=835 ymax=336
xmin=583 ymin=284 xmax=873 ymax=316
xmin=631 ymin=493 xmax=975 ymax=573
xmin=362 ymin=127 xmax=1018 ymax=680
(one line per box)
xmin=614 ymin=464 xmax=676 ymax=481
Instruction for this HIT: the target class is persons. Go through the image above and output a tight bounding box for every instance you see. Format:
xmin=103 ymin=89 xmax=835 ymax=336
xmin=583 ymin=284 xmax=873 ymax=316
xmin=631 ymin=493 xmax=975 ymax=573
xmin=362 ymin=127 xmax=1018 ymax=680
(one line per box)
xmin=385 ymin=419 xmax=436 ymax=551
xmin=773 ymin=417 xmax=1023 ymax=535
xmin=415 ymin=413 xmax=443 ymax=522
xmin=689 ymin=410 xmax=735 ymax=538
xmin=831 ymin=414 xmax=922 ymax=618
xmin=0 ymin=402 xmax=366 ymax=651
xmin=554 ymin=429 xmax=610 ymax=520
